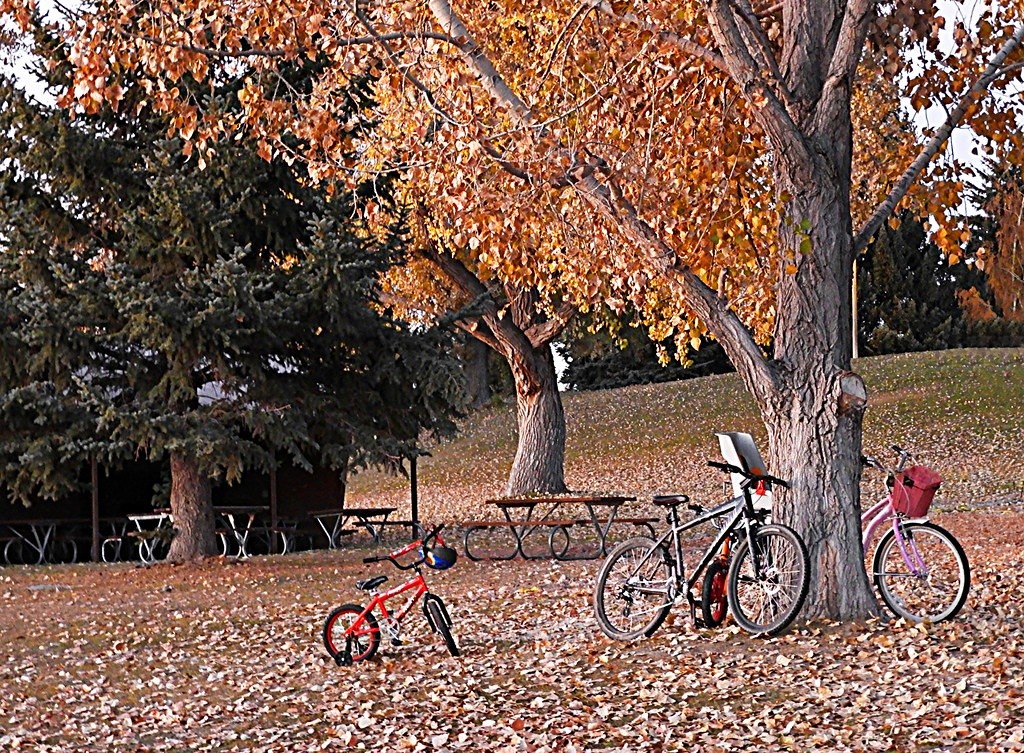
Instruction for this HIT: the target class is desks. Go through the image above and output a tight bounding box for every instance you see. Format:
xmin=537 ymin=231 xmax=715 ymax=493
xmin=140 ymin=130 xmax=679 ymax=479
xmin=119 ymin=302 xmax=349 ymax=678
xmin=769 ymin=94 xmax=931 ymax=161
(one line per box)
xmin=307 ymin=508 xmax=397 ymax=550
xmin=214 ymin=505 xmax=272 ymax=559
xmin=258 ymin=515 xmax=307 ymax=553
xmin=126 ymin=513 xmax=168 ymax=563
xmin=0 ymin=518 xmax=78 ymax=566
xmin=486 ymin=496 xmax=637 ymax=559
xmin=80 ymin=518 xmax=133 ymax=562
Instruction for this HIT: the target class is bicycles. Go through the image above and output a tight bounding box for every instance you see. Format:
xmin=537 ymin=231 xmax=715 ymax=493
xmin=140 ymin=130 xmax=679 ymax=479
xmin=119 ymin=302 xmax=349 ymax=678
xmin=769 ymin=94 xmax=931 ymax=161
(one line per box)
xmin=322 ymin=523 xmax=460 ymax=667
xmin=593 ymin=461 xmax=811 ymax=642
xmin=741 ymin=446 xmax=970 ymax=625
xmin=686 ymin=504 xmax=771 ymax=628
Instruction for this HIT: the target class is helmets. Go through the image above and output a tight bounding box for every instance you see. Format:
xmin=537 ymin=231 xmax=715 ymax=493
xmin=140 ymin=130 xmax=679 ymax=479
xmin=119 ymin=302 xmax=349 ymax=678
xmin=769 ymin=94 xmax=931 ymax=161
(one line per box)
xmin=418 ymin=547 xmax=458 ymax=571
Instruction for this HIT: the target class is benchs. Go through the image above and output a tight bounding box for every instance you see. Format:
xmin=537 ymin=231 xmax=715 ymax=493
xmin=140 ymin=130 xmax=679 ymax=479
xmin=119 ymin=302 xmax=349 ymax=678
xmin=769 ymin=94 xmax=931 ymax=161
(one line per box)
xmin=101 ymin=536 xmax=123 ymax=563
xmin=352 ymin=520 xmax=426 ymax=540
xmin=461 ymin=519 xmax=577 ymax=562
xmin=270 ymin=527 xmax=295 ymax=554
xmin=296 ymin=529 xmax=358 ymax=550
xmin=300 ymin=530 xmax=322 ymax=550
xmin=545 ymin=517 xmax=661 ymax=555
xmin=52 ymin=535 xmax=77 ymax=563
xmin=0 ymin=536 xmax=27 ymax=566
xmin=214 ymin=529 xmax=234 ymax=559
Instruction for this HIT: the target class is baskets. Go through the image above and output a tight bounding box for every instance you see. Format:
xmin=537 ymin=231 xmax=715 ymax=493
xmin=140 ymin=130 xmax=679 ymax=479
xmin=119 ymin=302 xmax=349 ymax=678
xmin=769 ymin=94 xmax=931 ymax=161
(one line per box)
xmin=891 ymin=465 xmax=943 ymax=517
